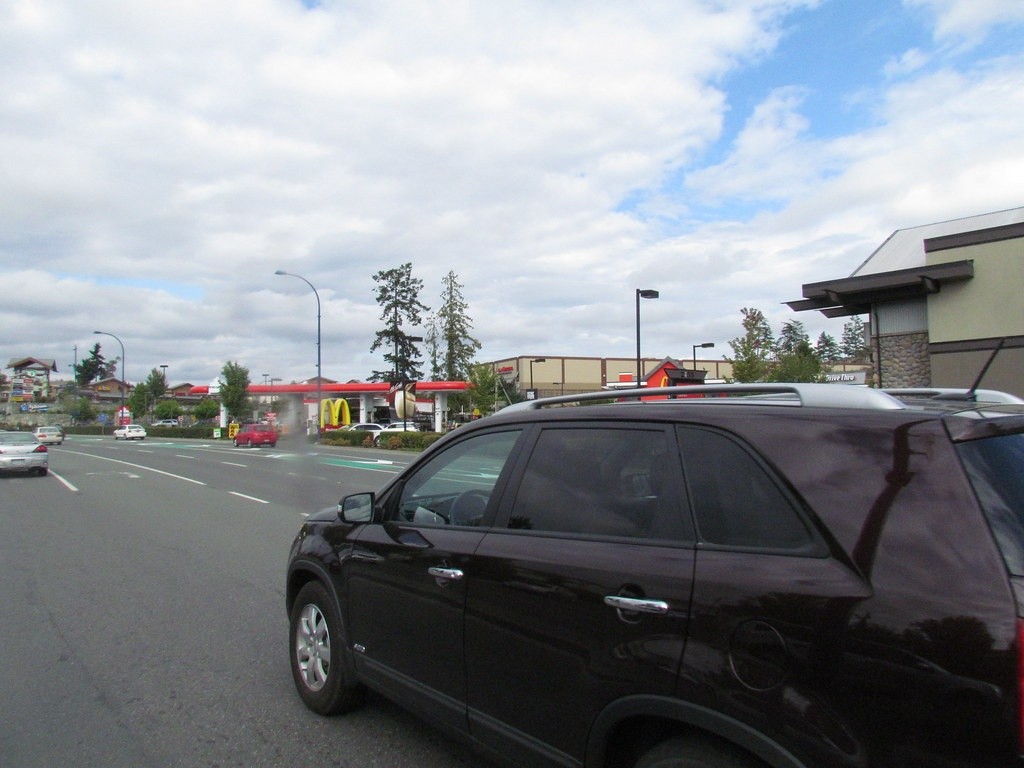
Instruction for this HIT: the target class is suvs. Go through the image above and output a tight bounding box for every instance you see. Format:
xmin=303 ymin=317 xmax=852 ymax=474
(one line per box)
xmin=286 ymin=381 xmax=1024 ymax=768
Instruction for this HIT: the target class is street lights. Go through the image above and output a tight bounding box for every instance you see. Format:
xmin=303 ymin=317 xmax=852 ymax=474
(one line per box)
xmin=530 ymin=359 xmax=546 ymax=389
xmin=262 ymin=374 xmax=269 ymax=400
xmin=275 ymin=270 xmax=321 ymax=442
xmin=160 ymin=364 xmax=168 ymax=376
xmin=402 ymin=336 xmax=423 ymax=431
xmin=637 ymin=288 xmax=659 ymax=401
xmin=270 ymin=378 xmax=282 ymax=403
xmin=693 ymin=343 xmax=714 ymax=370
xmin=94 ymin=331 xmax=124 ymax=425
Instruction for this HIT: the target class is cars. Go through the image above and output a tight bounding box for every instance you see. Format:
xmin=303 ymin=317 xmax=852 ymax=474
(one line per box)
xmin=31 ymin=423 xmax=65 ymax=446
xmin=0 ymin=430 xmax=50 ymax=476
xmin=374 ymin=421 xmax=428 ymax=448
xmin=188 ymin=418 xmax=220 ymax=428
xmin=233 ymin=417 xmax=319 ymax=448
xmin=338 ymin=422 xmax=387 ymax=433
xmin=151 ymin=418 xmax=179 ymax=428
xmin=113 ymin=424 xmax=147 ymax=440
